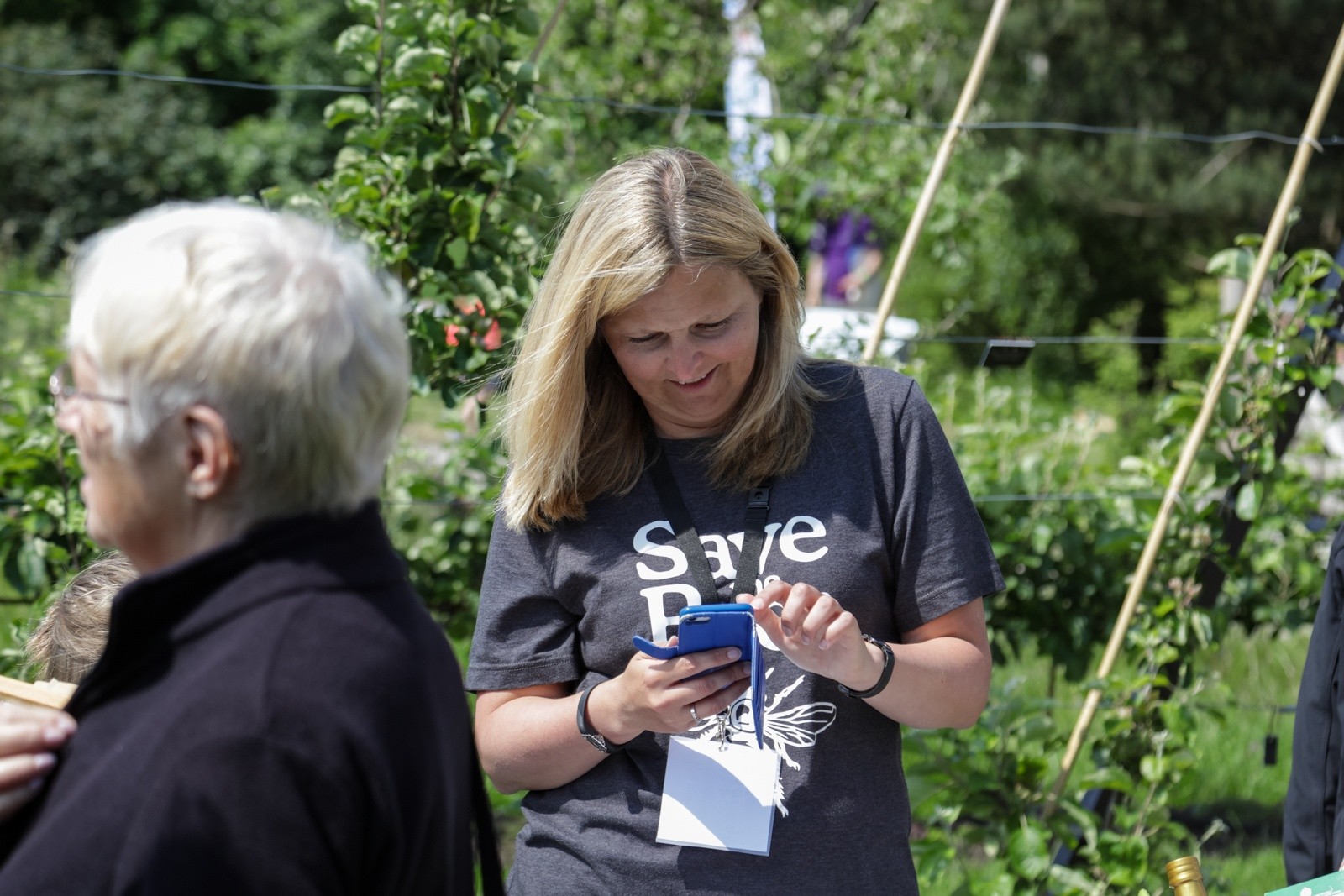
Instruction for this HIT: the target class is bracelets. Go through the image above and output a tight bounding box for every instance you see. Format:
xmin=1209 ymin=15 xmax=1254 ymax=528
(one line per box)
xmin=837 ymin=633 xmax=894 ymax=698
xmin=577 ymin=681 xmax=645 ymax=755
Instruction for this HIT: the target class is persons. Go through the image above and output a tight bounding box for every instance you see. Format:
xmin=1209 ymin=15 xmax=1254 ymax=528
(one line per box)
xmin=0 ymin=193 xmax=506 ymax=896
xmin=803 ymin=189 xmax=889 ymax=308
xmin=467 ymin=146 xmax=1009 ymax=896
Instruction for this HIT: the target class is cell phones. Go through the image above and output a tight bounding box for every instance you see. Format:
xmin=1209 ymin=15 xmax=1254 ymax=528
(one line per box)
xmin=677 ymin=604 xmax=753 ymax=717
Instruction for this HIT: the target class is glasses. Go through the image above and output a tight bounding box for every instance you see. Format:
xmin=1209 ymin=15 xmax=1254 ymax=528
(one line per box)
xmin=50 ymin=365 xmax=243 ymax=472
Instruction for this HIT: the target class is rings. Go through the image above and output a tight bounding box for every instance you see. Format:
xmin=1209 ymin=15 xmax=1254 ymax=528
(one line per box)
xmin=690 ymin=703 xmax=702 ymax=724
